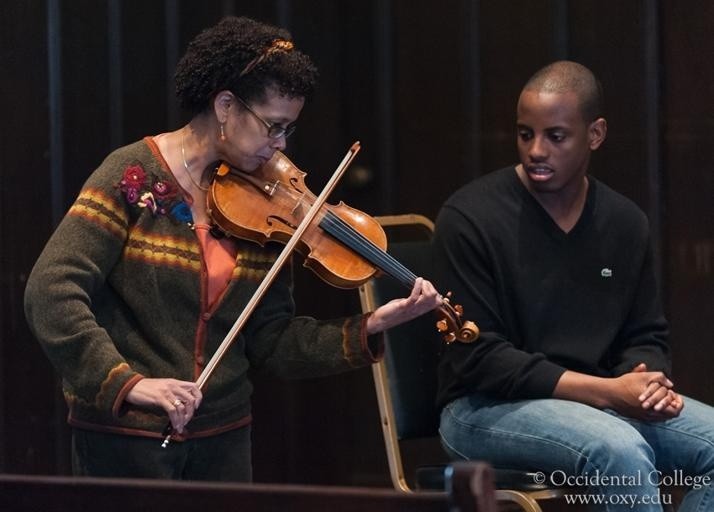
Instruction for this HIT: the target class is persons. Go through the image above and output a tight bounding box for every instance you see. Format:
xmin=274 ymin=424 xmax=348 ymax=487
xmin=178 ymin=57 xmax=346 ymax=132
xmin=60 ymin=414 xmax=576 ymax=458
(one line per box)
xmin=413 ymin=59 xmax=714 ymax=512
xmin=17 ymin=13 xmax=446 ymax=480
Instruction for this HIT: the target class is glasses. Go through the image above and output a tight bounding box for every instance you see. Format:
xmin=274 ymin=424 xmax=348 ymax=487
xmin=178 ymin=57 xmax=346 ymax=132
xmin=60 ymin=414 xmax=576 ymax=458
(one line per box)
xmin=233 ymin=93 xmax=297 ymax=140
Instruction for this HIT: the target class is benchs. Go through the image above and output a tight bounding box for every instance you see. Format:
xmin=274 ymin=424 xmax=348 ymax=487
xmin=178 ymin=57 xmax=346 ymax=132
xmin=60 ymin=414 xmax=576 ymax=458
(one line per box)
xmin=0 ymin=460 xmax=500 ymax=511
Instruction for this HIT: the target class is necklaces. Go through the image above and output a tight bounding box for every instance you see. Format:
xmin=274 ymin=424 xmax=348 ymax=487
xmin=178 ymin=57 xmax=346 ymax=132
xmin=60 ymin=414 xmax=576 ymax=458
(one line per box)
xmin=178 ymin=128 xmax=210 ymax=193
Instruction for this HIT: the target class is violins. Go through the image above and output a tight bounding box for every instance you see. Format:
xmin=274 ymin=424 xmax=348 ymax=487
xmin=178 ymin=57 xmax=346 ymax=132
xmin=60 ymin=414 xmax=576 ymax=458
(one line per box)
xmin=205 ymin=150 xmax=480 ymax=344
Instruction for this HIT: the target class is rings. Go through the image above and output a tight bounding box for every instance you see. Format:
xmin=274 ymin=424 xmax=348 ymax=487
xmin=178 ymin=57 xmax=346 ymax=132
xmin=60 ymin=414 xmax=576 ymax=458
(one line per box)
xmin=173 ymin=399 xmax=181 ymax=406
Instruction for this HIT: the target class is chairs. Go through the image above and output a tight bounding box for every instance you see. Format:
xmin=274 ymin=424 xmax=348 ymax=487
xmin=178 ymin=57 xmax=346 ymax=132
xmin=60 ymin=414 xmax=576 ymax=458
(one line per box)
xmin=355 ymin=213 xmax=588 ymax=512
